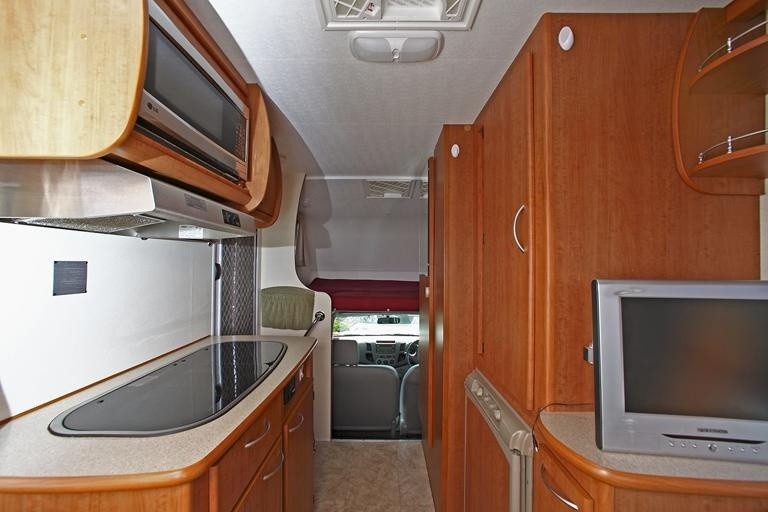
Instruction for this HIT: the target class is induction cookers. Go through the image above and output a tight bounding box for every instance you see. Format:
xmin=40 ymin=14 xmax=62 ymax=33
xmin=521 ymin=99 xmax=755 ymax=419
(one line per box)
xmin=50 ymin=339 xmax=288 ymax=437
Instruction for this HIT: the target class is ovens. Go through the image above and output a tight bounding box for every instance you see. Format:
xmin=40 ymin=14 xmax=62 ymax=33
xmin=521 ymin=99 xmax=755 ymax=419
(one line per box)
xmin=136 ymin=0 xmax=251 ymax=185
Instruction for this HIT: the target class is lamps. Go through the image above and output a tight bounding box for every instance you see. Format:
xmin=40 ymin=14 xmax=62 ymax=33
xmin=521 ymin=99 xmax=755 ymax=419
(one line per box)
xmin=348 ymin=31 xmax=445 ymax=64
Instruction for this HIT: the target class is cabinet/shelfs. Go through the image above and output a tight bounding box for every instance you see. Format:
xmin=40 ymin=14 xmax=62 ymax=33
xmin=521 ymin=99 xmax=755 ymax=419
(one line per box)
xmin=529 ymin=443 xmax=594 ymax=511
xmin=284 ymin=352 xmax=316 ymax=512
xmin=208 ymin=390 xmax=286 ymax=512
xmin=671 ymin=1 xmax=768 ymax=196
xmin=478 ymin=48 xmax=539 ymax=413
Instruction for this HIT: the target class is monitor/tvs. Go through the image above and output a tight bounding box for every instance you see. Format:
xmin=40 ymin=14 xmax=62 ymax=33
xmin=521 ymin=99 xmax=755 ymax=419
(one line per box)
xmin=589 ymin=279 xmax=768 ymax=466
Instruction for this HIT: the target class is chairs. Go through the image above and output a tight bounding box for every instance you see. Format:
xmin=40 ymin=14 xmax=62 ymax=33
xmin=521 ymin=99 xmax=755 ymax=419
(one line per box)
xmin=331 ymin=339 xmax=419 ymax=440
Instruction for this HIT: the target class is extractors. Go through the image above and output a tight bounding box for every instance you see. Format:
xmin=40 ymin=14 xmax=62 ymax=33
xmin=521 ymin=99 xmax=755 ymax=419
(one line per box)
xmin=0 ymin=157 xmax=257 ymax=241
